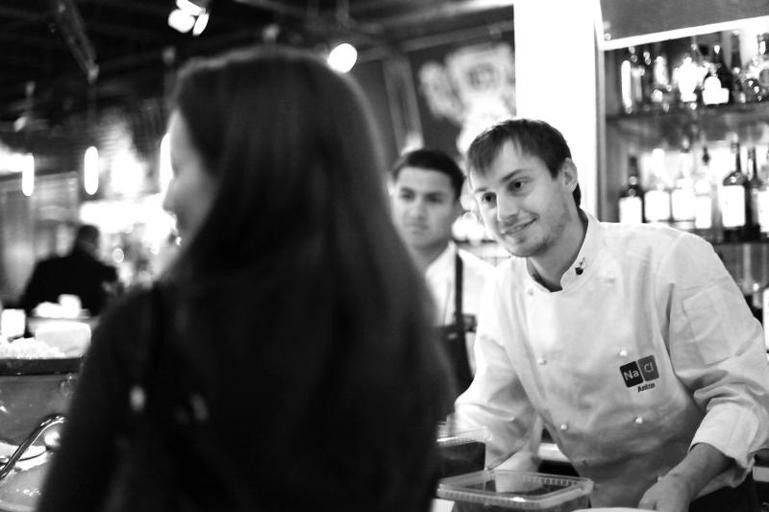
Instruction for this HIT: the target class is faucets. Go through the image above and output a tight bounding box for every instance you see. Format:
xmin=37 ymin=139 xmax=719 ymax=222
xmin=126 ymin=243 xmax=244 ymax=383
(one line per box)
xmin=0 ymin=413 xmax=69 ymax=485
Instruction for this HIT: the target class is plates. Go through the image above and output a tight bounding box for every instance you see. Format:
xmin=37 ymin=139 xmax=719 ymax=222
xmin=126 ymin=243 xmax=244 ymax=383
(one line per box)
xmin=0 ymin=353 xmax=84 ymax=377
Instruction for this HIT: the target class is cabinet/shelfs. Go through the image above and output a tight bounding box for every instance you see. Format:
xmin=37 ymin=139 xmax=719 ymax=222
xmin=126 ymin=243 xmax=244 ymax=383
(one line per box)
xmin=601 ymin=14 xmax=769 ymax=313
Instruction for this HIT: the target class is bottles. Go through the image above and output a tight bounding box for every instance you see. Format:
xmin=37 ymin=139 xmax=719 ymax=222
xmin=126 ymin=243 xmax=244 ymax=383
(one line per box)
xmin=615 ymin=134 xmax=769 ymax=243
xmin=616 ymin=30 xmax=768 ymax=115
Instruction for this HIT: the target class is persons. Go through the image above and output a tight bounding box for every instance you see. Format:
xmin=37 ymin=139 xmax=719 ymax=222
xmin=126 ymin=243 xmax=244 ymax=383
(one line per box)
xmin=33 ymin=47 xmax=455 ymax=512
xmin=445 ymin=118 xmax=769 ymax=511
xmin=19 ymin=224 xmax=113 ymax=312
xmin=389 ymin=149 xmax=493 ymax=386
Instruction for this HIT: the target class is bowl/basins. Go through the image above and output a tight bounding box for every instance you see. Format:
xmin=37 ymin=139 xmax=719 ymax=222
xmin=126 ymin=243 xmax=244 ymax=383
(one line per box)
xmin=24 ymin=317 xmax=91 ymax=348
